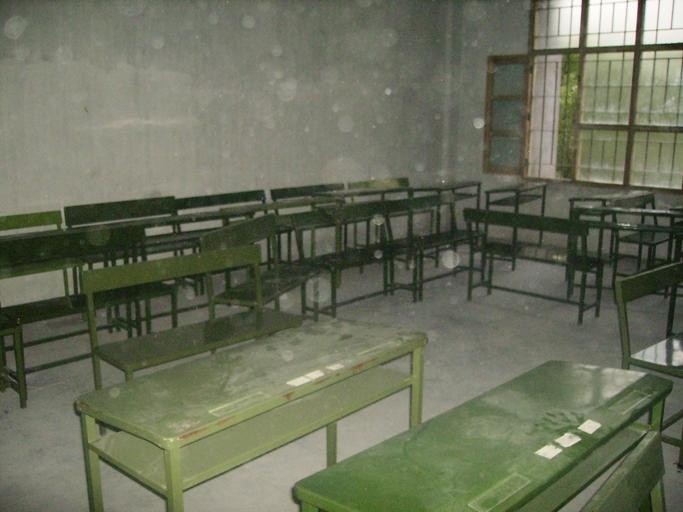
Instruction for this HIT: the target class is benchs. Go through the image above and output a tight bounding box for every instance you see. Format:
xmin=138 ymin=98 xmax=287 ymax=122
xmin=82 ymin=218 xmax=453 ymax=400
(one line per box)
xmin=462 ymin=207 xmax=607 ymax=324
xmin=579 ymin=429 xmax=668 ymax=511
xmin=614 ymin=261 xmax=683 ymax=470
xmin=1 ymin=177 xmax=480 ymax=409
xmin=565 ymin=191 xmax=683 ymax=299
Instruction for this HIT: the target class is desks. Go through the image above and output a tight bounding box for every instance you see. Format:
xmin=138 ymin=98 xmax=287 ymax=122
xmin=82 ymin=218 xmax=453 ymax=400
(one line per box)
xmin=291 ymin=360 xmax=674 ymax=511
xmin=481 ymin=182 xmax=548 ymax=271
xmin=73 ymin=316 xmax=428 ymax=512
